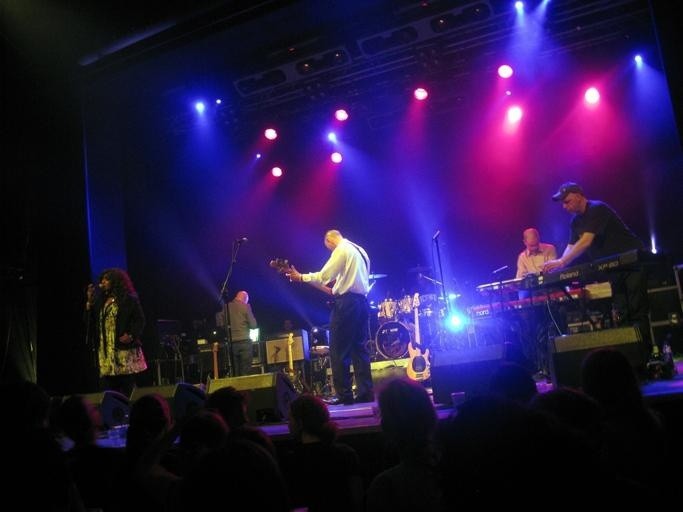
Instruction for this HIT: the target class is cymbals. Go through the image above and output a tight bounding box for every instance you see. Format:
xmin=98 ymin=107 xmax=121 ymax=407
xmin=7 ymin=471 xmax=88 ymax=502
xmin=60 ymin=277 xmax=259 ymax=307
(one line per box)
xmin=369 ymin=273 xmax=388 ymax=280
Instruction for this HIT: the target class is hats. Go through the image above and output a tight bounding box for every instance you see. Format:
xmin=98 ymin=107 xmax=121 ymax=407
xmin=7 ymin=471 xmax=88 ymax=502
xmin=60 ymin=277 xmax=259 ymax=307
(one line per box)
xmin=552 ymin=182 xmax=583 ymax=201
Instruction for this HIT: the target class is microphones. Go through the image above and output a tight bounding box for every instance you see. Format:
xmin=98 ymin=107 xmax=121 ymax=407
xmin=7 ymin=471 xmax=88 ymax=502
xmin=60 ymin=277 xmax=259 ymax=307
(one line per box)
xmin=236 ymin=235 xmax=250 ymax=247
xmin=431 ymin=227 xmax=442 ymax=243
xmin=88 ymin=284 xmax=105 ymax=290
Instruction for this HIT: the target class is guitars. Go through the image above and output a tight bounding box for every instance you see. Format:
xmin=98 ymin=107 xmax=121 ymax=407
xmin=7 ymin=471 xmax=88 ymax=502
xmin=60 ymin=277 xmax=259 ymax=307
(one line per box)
xmin=270 ymin=257 xmax=334 ymax=310
xmin=282 ymin=332 xmax=304 ymax=395
xmin=407 ymin=293 xmax=431 ymax=381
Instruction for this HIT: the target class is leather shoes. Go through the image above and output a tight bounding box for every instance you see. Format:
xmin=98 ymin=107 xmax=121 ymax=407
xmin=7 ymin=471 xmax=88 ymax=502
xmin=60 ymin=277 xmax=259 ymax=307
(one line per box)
xmin=353 ymin=394 xmax=375 ymax=404
xmin=322 ymin=394 xmax=354 ymax=405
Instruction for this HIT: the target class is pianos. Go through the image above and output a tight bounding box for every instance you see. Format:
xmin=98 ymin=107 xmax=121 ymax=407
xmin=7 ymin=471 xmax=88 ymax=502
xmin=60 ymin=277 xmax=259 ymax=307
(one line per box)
xmin=520 ymin=249 xmax=639 ymax=298
xmin=470 ymin=280 xmax=616 ymax=321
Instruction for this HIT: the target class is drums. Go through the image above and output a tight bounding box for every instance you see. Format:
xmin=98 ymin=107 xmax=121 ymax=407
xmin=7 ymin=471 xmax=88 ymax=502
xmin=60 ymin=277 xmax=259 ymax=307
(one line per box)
xmin=378 ymin=299 xmax=400 ymax=318
xmin=375 ymin=320 xmax=414 ymax=359
xmin=312 ymin=329 xmax=331 ymax=353
xmin=399 ymin=295 xmax=414 ymax=314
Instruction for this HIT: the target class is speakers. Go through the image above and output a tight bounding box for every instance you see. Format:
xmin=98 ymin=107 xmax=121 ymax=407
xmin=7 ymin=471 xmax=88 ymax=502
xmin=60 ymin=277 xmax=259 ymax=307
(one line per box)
xmin=430 ymin=342 xmax=518 ymax=405
xmin=59 ymin=391 xmax=130 ymax=430
xmin=204 ymin=372 xmax=296 ymax=425
xmin=129 ymin=383 xmax=208 ymax=425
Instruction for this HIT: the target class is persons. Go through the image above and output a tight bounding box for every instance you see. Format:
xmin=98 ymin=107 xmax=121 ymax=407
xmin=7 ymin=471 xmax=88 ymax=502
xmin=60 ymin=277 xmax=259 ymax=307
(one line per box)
xmin=222 ymin=290 xmax=257 ymax=378
xmin=82 ymin=268 xmax=146 ymax=399
xmin=288 ymin=228 xmax=375 ymax=406
xmin=0 ymin=379 xmax=683 ymax=511
xmin=516 ymin=228 xmax=556 ymax=300
xmin=541 ymin=182 xmax=653 ymax=383
